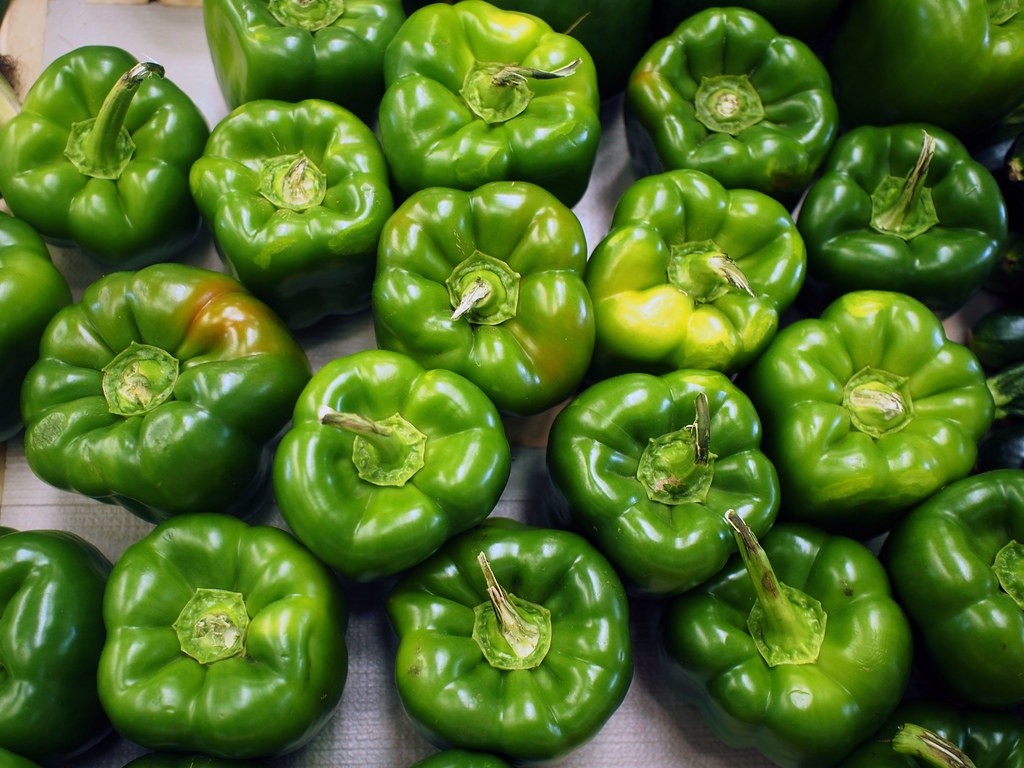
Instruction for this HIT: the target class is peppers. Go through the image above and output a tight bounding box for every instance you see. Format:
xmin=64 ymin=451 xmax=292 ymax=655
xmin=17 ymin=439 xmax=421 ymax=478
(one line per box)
xmin=0 ymin=0 xmax=1024 ymax=768
